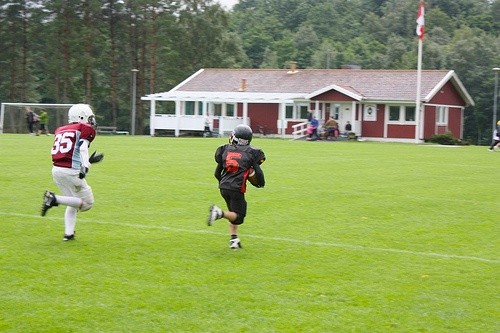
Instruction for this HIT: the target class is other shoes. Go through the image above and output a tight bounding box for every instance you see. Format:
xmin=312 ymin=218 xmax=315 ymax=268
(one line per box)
xmin=207 ymin=204 xmax=222 ymax=226
xmin=229 ymin=238 xmax=242 ymax=249
xmin=40 ymin=190 xmax=59 ymax=217
xmin=64 ymin=231 xmax=76 ymax=241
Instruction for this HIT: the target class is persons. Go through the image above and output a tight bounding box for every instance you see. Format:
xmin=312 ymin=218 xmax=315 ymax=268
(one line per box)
xmin=41 ymin=103 xmax=103 ymax=241
xmin=324 ymin=116 xmax=337 ymax=140
xmin=488 ymin=121 xmax=500 ymax=151
xmin=24 ymin=106 xmax=48 ymax=136
xmin=208 ymin=124 xmax=266 ymax=248
xmin=309 ymin=116 xmax=320 ymax=138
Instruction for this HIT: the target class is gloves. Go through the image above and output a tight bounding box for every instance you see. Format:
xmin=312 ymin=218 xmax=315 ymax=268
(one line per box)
xmin=89 ymin=151 xmax=103 ymax=163
xmin=79 ymin=173 xmax=85 ymax=179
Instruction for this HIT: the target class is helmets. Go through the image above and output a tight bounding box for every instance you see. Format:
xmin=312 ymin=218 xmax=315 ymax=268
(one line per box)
xmin=229 ymin=125 xmax=253 ymax=146
xmin=68 ymin=103 xmax=97 ymax=129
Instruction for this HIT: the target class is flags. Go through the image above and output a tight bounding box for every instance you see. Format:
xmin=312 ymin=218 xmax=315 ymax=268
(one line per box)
xmin=416 ymin=0 xmax=425 ymax=39
